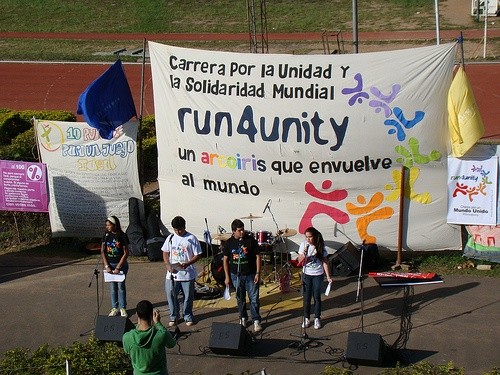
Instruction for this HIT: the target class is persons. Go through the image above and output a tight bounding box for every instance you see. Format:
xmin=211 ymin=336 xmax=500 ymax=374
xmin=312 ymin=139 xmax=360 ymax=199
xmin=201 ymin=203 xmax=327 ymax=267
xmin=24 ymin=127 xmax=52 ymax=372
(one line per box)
xmin=298 ymin=226 xmax=332 ymax=329
xmin=122 ymin=300 xmax=176 ymax=375
xmin=223 ymin=218 xmax=263 ymax=332
xmin=161 ymin=215 xmax=203 ymax=326
xmin=101 ymin=215 xmax=130 ymax=318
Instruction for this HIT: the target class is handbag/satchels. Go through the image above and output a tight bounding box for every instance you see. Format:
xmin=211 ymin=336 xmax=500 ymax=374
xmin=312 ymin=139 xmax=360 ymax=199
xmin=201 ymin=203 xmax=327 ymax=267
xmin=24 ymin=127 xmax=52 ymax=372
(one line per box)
xmin=224 ymin=284 xmax=231 ymax=300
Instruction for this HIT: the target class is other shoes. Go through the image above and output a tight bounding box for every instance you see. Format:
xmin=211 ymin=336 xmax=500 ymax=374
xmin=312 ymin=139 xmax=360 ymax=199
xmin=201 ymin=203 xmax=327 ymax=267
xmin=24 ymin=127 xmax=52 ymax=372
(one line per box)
xmin=169 ymin=318 xmax=177 ymax=326
xmin=184 ymin=316 xmax=193 ymax=326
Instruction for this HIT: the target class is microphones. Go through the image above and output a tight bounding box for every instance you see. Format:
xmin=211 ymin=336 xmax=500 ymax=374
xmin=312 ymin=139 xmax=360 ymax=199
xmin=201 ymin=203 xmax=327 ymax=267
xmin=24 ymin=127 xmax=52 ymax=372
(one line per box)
xmin=305 ymin=244 xmax=309 ymax=255
xmin=334 ymin=223 xmax=337 ymax=236
xmin=105 ymin=233 xmax=109 ymax=242
xmin=263 ymin=200 xmax=270 ymax=213
xmin=169 ymin=234 xmax=174 ymax=242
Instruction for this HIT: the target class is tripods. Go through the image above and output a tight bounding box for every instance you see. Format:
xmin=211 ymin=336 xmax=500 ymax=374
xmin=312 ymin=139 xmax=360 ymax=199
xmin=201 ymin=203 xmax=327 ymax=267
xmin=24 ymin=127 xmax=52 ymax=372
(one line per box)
xmin=287 ymin=252 xmax=328 ymax=347
xmin=80 ymin=244 xmax=106 ymax=336
xmin=168 ymin=242 xmax=199 ymax=340
xmin=204 ymin=208 xmax=301 ymax=294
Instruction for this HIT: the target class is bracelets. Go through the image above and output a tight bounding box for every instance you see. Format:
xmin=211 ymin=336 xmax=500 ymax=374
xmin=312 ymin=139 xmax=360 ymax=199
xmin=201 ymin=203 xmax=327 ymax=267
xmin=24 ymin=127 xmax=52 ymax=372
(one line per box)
xmin=256 ymin=271 xmax=260 ymax=274
xmin=105 ymin=264 xmax=110 ymax=268
xmin=115 ymin=267 xmax=120 ymax=270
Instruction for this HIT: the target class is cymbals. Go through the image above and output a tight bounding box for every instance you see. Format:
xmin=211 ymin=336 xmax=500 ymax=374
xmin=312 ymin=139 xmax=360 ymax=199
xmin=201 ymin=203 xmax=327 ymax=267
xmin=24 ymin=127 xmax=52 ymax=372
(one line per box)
xmin=240 ymin=216 xmax=263 ymax=219
xmin=279 ymin=229 xmax=297 ymax=237
xmin=212 ymin=233 xmax=232 ymax=241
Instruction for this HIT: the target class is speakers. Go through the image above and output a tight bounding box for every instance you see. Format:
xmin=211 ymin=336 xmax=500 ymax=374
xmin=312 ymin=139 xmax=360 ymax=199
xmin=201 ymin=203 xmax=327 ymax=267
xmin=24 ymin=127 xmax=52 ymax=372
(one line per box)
xmin=345 ymin=331 xmax=385 ymax=365
xmin=208 ymin=322 xmax=252 ymax=356
xmin=329 ymin=241 xmax=360 ymax=277
xmin=94 ymin=315 xmax=136 ymax=345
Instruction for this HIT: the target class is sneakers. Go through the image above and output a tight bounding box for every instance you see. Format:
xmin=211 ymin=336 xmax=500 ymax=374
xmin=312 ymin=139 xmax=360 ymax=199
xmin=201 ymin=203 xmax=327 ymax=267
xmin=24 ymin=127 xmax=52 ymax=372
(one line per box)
xmin=239 ymin=318 xmax=248 ymax=327
xmin=301 ymin=317 xmax=310 ymax=328
xmin=108 ymin=308 xmax=119 ymax=316
xmin=120 ymin=308 xmax=128 ymax=317
xmin=314 ymin=318 xmax=321 ymax=329
xmin=254 ymin=320 xmax=262 ymax=331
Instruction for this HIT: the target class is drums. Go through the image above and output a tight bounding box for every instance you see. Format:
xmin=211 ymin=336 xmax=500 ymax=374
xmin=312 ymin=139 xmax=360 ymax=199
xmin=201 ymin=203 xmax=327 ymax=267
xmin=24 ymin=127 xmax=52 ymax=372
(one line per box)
xmin=256 ymin=231 xmax=272 ymax=247
xmin=211 ymin=253 xmax=234 ymax=288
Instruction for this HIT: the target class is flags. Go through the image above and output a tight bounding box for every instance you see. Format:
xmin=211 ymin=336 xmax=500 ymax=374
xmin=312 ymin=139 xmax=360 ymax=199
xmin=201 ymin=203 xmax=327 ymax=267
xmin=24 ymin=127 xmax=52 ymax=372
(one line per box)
xmin=447 ymin=66 xmax=485 ymax=157
xmin=76 ymin=59 xmax=138 ymax=139
